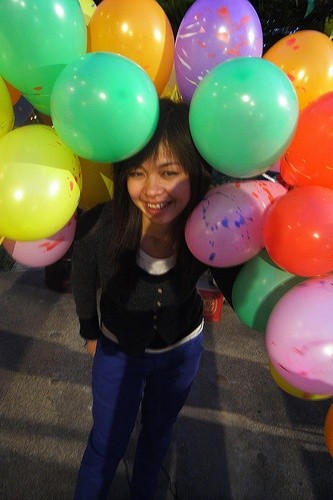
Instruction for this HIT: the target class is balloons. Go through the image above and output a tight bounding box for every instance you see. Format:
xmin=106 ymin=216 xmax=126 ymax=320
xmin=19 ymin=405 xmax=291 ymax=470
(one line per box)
xmin=0 ymin=0 xmax=333 ymax=452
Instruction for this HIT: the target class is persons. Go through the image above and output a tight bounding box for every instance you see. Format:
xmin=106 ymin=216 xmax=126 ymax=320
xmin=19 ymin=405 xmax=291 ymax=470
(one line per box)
xmin=69 ymin=98 xmax=244 ymax=500
